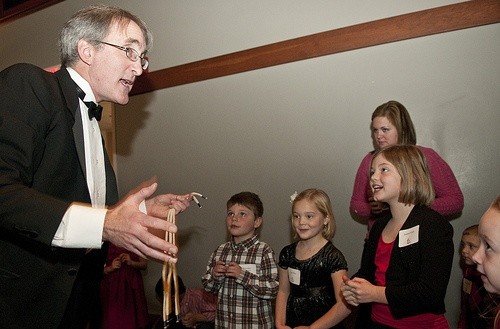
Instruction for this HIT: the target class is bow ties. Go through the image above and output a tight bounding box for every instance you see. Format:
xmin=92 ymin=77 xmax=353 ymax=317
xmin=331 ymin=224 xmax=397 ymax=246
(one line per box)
xmin=80 ymin=97 xmax=104 ymax=121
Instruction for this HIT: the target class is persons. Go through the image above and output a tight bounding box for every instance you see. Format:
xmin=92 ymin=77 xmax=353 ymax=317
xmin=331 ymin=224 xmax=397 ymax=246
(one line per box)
xmin=0 ymin=3 xmax=193 ymax=329
xmin=456 ymin=195 xmax=500 ymax=329
xmin=104 ymin=240 xmax=150 ymax=329
xmin=201 ymin=192 xmax=280 ymax=329
xmin=340 ymin=144 xmax=454 ymax=329
xmin=274 ymin=189 xmax=352 ymax=329
xmin=350 ymin=100 xmax=464 ymax=243
xmin=155 ymin=273 xmax=218 ymax=329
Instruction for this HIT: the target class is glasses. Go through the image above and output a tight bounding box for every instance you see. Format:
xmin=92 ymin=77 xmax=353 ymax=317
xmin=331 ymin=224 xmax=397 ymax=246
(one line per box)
xmin=93 ymin=39 xmax=149 ymax=70
